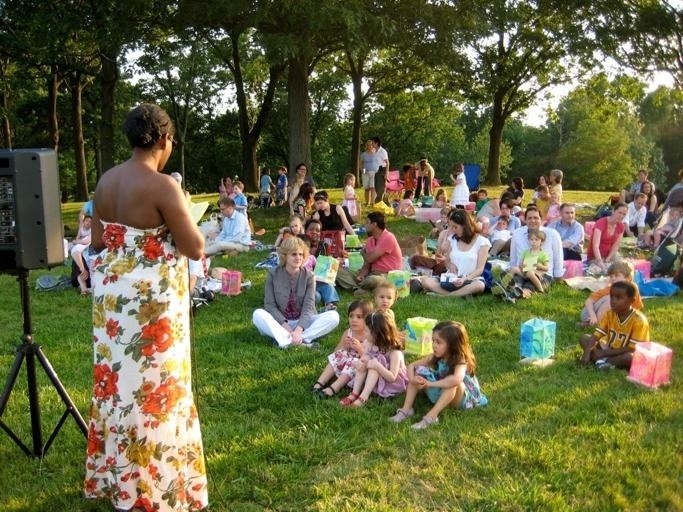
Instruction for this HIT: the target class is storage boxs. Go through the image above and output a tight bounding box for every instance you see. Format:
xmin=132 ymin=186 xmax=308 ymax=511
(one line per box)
xmin=397 ymin=234 xmax=419 ymax=259
xmin=409 ymin=236 xmax=429 ymax=257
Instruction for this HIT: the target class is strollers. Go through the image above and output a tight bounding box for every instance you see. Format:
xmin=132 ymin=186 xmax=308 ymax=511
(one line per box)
xmin=649 ymin=180 xmax=682 ymax=279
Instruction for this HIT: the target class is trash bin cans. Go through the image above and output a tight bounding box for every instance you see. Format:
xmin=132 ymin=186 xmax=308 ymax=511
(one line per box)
xmin=464 ymin=163 xmax=480 ymax=192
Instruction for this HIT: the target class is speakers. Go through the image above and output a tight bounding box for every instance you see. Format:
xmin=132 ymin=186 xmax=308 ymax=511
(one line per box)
xmin=0 ymin=148 xmax=65 ymax=272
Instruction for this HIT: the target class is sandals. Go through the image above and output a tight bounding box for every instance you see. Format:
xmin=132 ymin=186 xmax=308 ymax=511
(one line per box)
xmin=325 ymin=302 xmax=337 ymax=311
xmin=310 ymin=381 xmax=325 ymax=393
xmin=318 ymin=386 xmax=337 ymax=400
xmin=351 ymin=397 xmax=368 ymax=408
xmin=337 ymin=392 xmax=360 ymax=406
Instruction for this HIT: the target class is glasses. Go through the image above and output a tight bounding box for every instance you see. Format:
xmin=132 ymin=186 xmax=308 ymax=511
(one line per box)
xmin=168 ymin=135 xmax=179 ymax=148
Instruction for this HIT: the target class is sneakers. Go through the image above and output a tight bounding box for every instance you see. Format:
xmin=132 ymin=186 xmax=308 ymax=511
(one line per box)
xmin=388 ymin=406 xmax=415 ymax=422
xmin=595 ymin=358 xmax=613 ymax=369
xmin=353 ymin=288 xmax=371 ymax=297
xmin=410 ymin=416 xmax=439 ymax=430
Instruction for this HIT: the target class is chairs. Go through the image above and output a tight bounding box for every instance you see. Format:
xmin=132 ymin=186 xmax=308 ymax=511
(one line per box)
xmin=380 ymin=169 xmax=405 ymax=200
xmin=461 ymin=163 xmax=481 ymax=194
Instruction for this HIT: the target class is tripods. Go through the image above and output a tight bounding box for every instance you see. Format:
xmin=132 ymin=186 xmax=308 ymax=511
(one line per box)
xmin=0 ymin=269 xmax=88 ymax=460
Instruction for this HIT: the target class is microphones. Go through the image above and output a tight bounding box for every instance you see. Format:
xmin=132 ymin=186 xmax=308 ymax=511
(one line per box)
xmin=171 ymin=172 xmax=183 ymax=185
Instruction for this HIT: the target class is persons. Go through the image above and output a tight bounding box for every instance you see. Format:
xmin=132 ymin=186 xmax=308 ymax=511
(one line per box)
xmin=85 ymin=101 xmax=206 ymax=512
xmin=310 ymin=298 xmax=377 ymax=400
xmin=577 ymin=279 xmax=652 ymax=372
xmin=61 ymin=136 xmax=683 ymax=349
xmin=339 ymin=307 xmax=409 ymax=410
xmin=388 ymin=318 xmax=489 ymax=432
